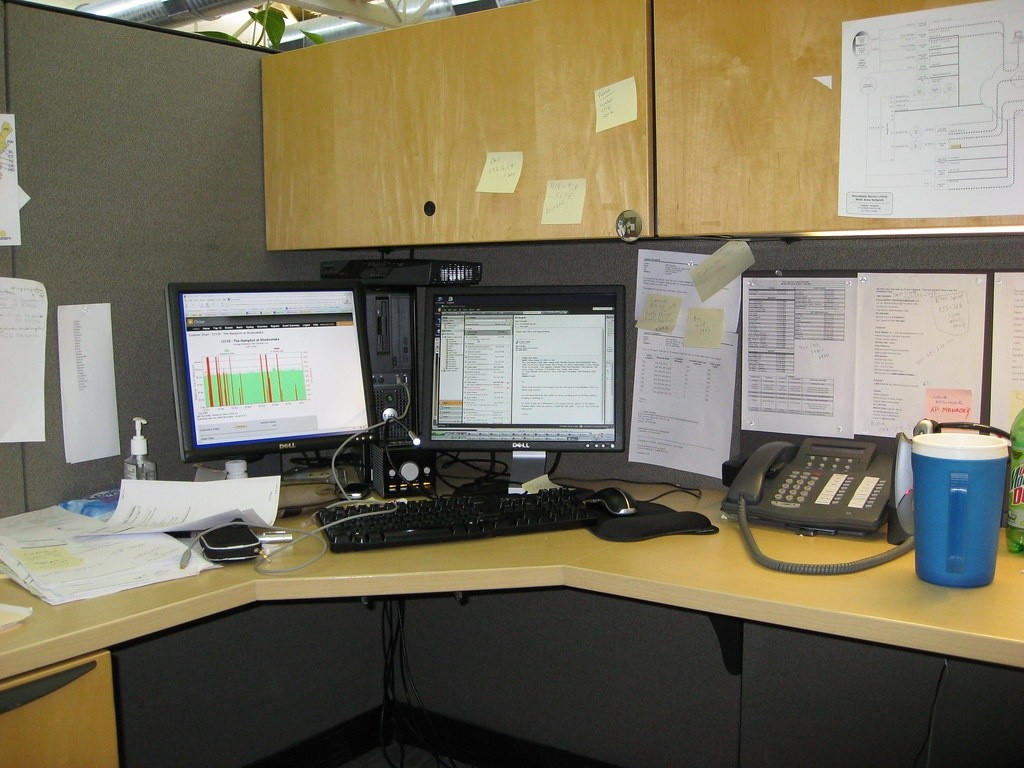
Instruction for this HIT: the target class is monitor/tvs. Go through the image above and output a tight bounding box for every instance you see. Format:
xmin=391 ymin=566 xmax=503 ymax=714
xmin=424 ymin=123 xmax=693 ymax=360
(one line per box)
xmin=165 ymin=279 xmax=378 ymax=480
xmin=420 ymin=285 xmax=627 ymax=494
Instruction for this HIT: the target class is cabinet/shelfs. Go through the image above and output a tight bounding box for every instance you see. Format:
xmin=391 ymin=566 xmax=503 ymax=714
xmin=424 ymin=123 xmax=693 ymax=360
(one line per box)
xmin=261 ymin=0 xmax=655 ymax=252
xmin=653 ymin=0 xmax=1024 ymax=239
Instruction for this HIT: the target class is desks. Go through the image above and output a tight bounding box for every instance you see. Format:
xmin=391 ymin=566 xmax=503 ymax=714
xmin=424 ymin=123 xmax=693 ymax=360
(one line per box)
xmin=0 ymin=466 xmax=1024 ymax=768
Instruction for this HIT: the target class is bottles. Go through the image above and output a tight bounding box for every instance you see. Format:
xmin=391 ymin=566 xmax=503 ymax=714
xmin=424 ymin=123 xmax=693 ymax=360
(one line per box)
xmin=225 ymin=460 xmax=248 ymax=480
xmin=1006 ymin=408 xmax=1024 ymax=555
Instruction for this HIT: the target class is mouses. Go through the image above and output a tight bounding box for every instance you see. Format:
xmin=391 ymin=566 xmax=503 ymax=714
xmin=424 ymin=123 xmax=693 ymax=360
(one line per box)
xmin=582 ymin=487 xmax=639 ymax=517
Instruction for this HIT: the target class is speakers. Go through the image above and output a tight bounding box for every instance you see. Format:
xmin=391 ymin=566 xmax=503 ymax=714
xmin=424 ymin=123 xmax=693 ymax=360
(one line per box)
xmin=886 ymin=432 xmax=915 ymax=546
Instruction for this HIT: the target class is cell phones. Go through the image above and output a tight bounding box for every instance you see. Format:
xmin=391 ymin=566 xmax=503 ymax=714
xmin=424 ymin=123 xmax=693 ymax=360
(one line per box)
xmin=337 ymin=483 xmax=371 ymax=500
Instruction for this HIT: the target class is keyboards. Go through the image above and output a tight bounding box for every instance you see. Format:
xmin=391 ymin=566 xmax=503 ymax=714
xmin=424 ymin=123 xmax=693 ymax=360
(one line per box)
xmin=316 ymin=487 xmax=598 ymax=553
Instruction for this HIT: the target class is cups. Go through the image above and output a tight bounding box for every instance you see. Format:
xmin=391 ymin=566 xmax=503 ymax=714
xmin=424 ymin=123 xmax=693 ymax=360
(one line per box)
xmin=912 ymin=434 xmax=1009 ymax=588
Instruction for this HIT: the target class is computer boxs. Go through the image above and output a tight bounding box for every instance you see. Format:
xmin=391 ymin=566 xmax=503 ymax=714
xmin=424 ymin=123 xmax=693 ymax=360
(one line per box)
xmin=361 ymin=289 xmax=420 ymax=446
